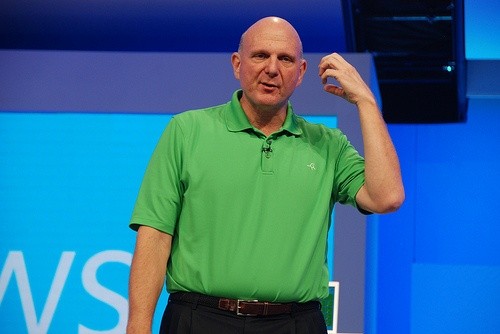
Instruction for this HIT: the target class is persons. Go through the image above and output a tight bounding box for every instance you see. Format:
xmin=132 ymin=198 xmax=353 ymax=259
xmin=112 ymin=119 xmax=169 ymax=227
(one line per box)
xmin=126 ymin=15 xmax=406 ymax=333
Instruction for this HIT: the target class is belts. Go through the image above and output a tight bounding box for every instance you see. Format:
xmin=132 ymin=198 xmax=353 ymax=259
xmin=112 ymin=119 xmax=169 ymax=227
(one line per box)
xmin=168 ymin=291 xmax=320 ymax=317
